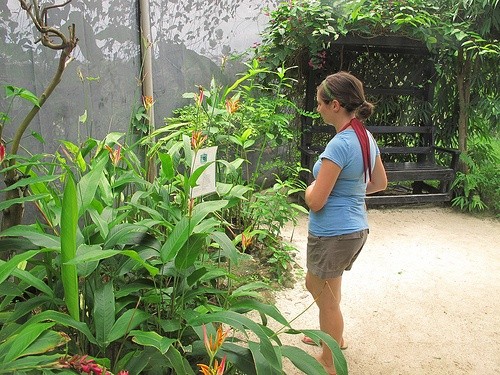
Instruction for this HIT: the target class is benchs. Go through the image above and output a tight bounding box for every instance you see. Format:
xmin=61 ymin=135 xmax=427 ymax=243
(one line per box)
xmin=298 ymin=123 xmax=461 ymax=212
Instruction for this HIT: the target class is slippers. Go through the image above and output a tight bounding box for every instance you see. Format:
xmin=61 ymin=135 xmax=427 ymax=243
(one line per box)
xmin=302 ymin=336 xmax=348 ymax=349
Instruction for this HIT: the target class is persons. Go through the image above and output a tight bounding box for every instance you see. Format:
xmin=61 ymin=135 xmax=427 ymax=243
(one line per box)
xmin=301 ymin=70 xmax=388 ymax=375
